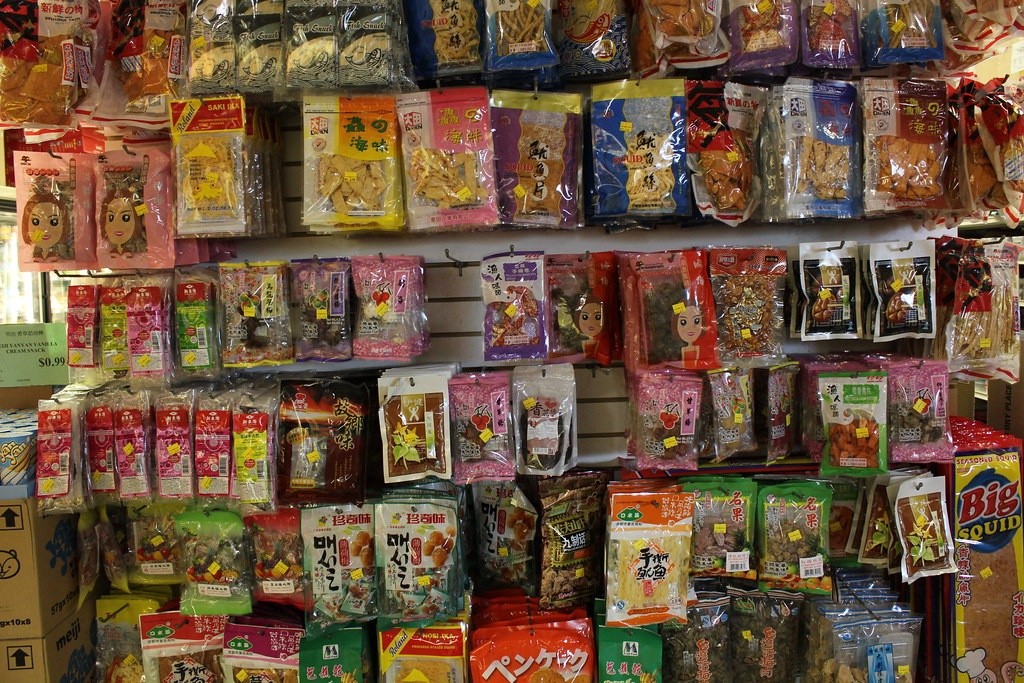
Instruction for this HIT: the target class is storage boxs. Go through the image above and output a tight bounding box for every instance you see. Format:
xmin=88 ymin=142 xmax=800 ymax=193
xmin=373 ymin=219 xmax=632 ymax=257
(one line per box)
xmin=0 ymin=596 xmax=96 ymax=683
xmin=0 ymin=496 xmax=82 ymax=640
